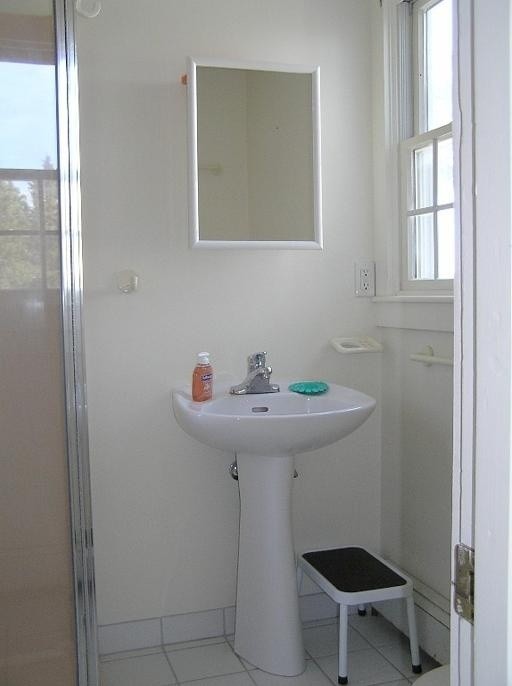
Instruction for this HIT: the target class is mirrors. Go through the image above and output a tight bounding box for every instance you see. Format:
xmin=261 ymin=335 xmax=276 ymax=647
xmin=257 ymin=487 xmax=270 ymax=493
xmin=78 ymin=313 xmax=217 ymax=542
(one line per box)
xmin=187 ymin=56 xmax=324 ymax=250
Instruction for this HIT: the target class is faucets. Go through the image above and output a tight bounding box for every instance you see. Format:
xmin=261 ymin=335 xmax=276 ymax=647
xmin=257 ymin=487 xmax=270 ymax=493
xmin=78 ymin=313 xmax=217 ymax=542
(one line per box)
xmin=228 ymin=350 xmax=281 ymax=394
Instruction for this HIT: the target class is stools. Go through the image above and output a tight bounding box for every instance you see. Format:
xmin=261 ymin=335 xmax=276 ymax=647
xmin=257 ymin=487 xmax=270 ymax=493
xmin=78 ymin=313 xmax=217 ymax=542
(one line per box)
xmin=297 ymin=544 xmax=423 ymax=685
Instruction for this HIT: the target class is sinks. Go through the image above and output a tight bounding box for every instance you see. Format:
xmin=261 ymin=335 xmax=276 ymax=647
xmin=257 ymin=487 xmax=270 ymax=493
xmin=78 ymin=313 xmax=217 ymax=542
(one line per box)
xmin=170 ymin=379 xmax=379 ymax=456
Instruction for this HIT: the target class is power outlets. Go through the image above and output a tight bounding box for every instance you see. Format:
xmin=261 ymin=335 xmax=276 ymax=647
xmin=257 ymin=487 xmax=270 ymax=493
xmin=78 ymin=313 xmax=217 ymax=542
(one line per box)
xmin=356 ymin=262 xmax=376 ymax=298
